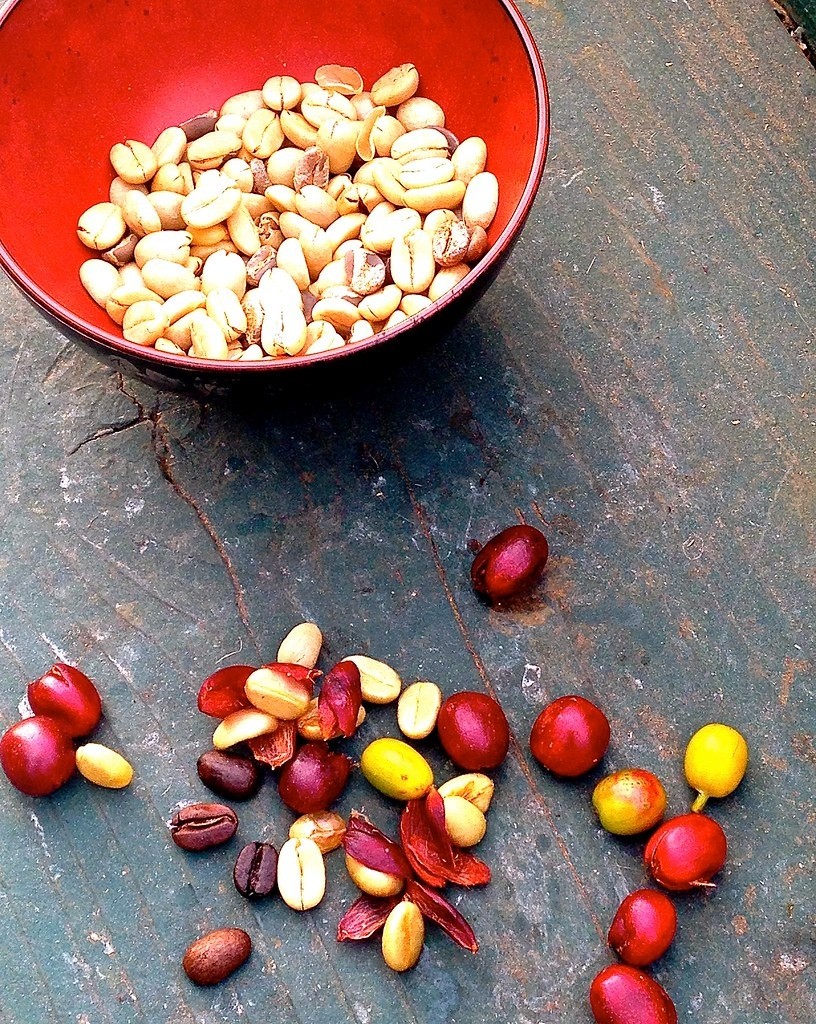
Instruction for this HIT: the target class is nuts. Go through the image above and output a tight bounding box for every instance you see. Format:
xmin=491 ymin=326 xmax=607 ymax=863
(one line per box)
xmin=77 ymin=64 xmax=500 ymax=362
xmin=77 ymin=621 xmax=491 ymax=983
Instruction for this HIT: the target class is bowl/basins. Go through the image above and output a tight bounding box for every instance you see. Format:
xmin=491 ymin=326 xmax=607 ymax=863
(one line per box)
xmin=0 ymin=0 xmax=551 ymax=416
